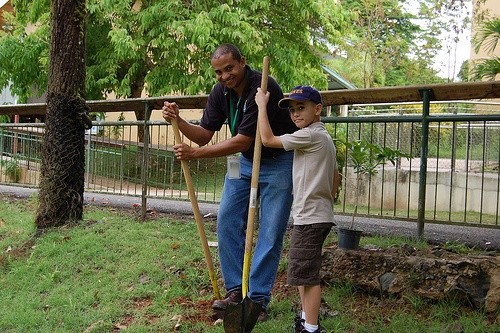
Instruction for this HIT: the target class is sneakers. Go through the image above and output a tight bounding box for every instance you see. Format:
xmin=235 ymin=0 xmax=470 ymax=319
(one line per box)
xmin=211 ymin=291 xmax=242 ymax=310
xmin=257 ymin=311 xmax=267 ymax=321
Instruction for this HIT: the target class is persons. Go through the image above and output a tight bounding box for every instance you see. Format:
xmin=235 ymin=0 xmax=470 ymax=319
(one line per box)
xmin=160 ymin=42 xmax=302 ymax=322
xmin=254 ymin=84 xmax=343 ymax=333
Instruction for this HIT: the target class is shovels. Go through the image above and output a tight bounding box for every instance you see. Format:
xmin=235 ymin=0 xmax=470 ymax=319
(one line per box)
xmin=223 ymin=55 xmax=263 ymax=333
xmin=166 ymin=108 xmax=229 ymax=322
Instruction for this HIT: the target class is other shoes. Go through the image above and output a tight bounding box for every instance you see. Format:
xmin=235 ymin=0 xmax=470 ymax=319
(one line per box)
xmin=294 ymin=324 xmax=320 ymax=333
xmin=294 ymin=315 xmax=319 ymax=330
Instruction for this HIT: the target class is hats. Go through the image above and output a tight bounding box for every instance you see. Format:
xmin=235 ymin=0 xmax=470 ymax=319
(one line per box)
xmin=278 ymin=86 xmax=321 ymax=109
